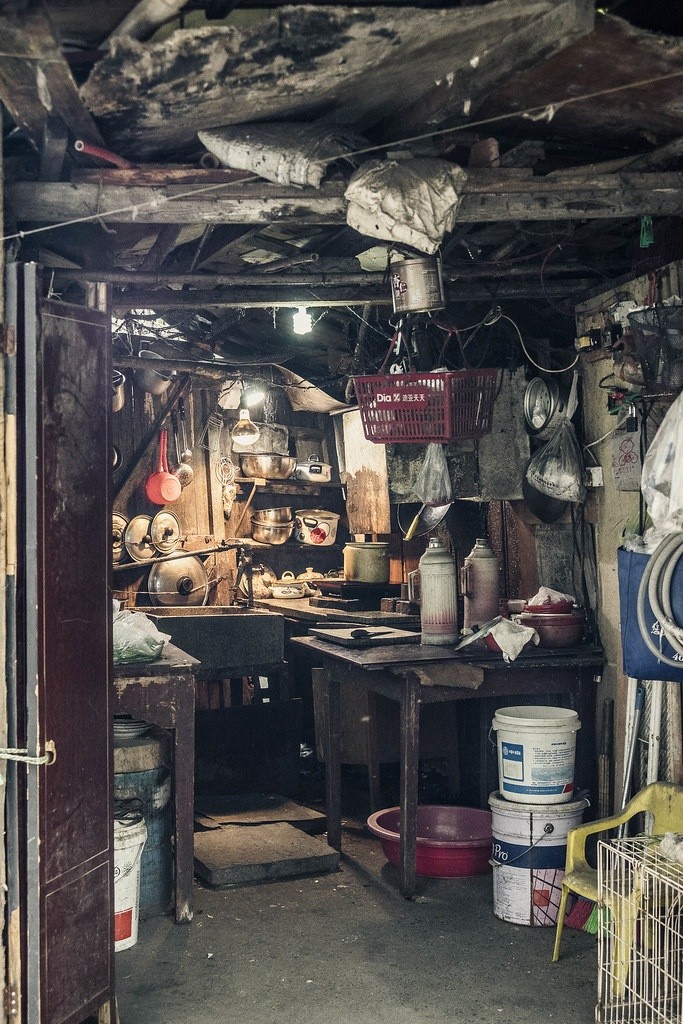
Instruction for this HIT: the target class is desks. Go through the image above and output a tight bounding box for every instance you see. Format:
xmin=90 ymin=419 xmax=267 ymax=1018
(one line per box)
xmin=111 ymin=640 xmax=202 ymax=927
xmin=289 ymin=632 xmax=609 ymax=900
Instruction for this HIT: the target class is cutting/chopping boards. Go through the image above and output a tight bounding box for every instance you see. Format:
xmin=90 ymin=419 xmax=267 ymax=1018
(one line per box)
xmin=307 ymin=622 xmax=423 ymax=648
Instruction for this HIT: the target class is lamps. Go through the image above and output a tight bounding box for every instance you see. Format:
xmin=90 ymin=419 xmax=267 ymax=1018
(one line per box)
xmin=293 ymin=307 xmax=313 ymax=335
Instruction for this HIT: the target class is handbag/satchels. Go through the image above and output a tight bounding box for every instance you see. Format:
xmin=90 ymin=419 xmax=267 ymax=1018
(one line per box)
xmin=527 ymin=420 xmax=590 ymax=502
xmin=413 ymin=441 xmax=456 ymax=508
xmin=618 ymin=529 xmax=683 ymax=684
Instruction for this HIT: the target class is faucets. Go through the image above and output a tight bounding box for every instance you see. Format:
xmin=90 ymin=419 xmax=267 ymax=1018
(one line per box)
xmin=227 ymin=556 xmax=253 ymax=593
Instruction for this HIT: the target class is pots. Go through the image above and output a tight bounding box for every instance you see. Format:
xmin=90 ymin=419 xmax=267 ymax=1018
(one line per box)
xmin=294 ymin=507 xmax=341 ymax=546
xmin=397 ymin=500 xmax=453 ymax=538
xmin=342 ymin=541 xmax=392 ymax=584
xmin=268 ymin=564 xmax=343 ymax=599
xmin=112 ymin=369 xmax=125 ymax=413
xmin=135 ymin=340 xmax=182 ymax=395
xmin=294 ymin=454 xmax=334 ymax=482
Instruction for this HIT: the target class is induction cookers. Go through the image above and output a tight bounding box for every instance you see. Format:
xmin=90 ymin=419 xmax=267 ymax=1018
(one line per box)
xmin=311 ymin=579 xmax=407 ymax=600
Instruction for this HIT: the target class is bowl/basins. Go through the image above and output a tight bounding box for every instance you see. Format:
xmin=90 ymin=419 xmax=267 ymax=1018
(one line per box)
xmin=240 ymin=452 xmax=297 ymax=480
xmin=482 ymin=634 xmax=503 ymax=652
xmin=367 ymin=805 xmax=492 ymax=879
xmin=518 ymin=600 xmax=588 ymax=649
xmin=250 ymin=505 xmax=295 ymax=544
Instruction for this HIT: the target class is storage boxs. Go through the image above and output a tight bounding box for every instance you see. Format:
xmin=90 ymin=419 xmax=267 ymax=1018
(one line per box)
xmin=351 ymin=366 xmax=500 ymax=445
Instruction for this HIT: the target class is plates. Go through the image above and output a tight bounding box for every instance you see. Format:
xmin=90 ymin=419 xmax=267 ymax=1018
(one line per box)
xmin=113 ymin=719 xmax=156 ymax=739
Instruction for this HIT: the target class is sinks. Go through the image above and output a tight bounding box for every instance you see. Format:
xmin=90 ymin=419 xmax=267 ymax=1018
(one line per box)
xmin=120 ymin=603 xmax=286 ymax=670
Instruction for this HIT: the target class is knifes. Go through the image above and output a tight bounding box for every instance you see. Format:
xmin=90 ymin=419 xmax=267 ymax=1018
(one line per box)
xmin=188 ymin=392 xmax=195 ymax=448
xmin=315 ymin=620 xmax=372 ymax=628
xmin=349 ymin=627 xmax=396 ymax=638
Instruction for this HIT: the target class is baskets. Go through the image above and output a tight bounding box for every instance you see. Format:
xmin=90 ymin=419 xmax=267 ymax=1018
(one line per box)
xmin=348 ymin=324 xmax=495 ymax=445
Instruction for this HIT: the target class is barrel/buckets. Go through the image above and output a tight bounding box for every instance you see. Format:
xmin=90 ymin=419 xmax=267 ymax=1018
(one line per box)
xmin=488 ymin=788 xmax=591 ymax=929
xmin=388 ymin=241 xmax=446 ymax=313
xmin=113 ymin=806 xmax=177 ymax=952
xmin=488 ymin=706 xmax=582 ymax=804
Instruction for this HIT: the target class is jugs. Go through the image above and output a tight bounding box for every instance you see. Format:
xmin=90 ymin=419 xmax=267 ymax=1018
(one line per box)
xmin=407 ymin=537 xmax=458 ymax=646
xmin=460 ymin=538 xmax=500 ymax=638
